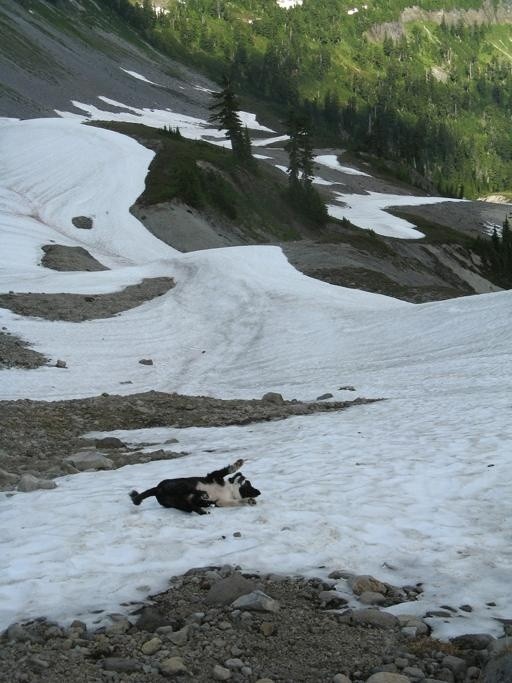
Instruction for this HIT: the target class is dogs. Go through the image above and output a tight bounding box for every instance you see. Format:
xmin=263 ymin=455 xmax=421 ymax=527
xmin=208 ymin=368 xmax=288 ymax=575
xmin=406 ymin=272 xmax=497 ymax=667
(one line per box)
xmin=130 ymin=459 xmax=260 ymax=515
